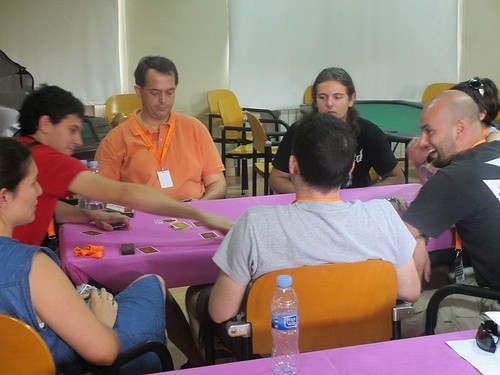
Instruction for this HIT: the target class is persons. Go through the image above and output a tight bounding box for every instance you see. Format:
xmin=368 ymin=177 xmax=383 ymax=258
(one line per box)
xmin=268 ymin=68 xmax=405 ymax=194
xmin=183 ymin=114 xmax=422 ymax=374
xmin=90 ymin=57 xmax=227 ymax=202
xmin=10 ymin=83 xmax=235 ymax=286
xmin=402 ymin=88 xmax=500 ymax=337
xmin=403 ymin=78 xmax=500 ymax=179
xmin=0 ymin=136 xmax=168 ymax=375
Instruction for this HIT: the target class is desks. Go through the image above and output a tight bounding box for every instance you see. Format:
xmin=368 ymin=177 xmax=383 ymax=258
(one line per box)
xmin=59 ymin=185 xmax=459 ymax=302
xmin=147 ymin=325 xmax=500 ymax=375
xmin=352 ymin=99 xmax=430 ymax=184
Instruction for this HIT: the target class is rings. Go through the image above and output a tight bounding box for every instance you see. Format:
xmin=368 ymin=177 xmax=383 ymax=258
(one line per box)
xmin=106 ymin=297 xmax=113 ymax=301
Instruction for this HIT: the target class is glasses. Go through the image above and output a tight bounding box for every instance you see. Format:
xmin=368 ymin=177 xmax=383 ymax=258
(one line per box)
xmin=476 ymin=320 xmax=498 ymax=353
xmin=469 ymin=77 xmax=484 ymax=96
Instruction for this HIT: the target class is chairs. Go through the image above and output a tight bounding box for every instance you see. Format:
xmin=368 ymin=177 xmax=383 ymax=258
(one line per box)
xmin=105 ymin=93 xmax=144 ymax=121
xmin=205 ymin=88 xmax=291 ymax=197
xmin=196 ymin=260 xmax=413 ymax=360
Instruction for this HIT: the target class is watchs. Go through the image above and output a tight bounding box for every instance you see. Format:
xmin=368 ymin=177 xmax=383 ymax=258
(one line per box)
xmin=384 ymin=197 xmax=401 ymax=204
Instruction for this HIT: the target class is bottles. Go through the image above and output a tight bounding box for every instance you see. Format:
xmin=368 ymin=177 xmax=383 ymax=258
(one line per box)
xmin=270 ymin=274 xmax=300 ymax=375
xmin=76 ymin=159 xmax=89 ymax=209
xmin=85 ymin=161 xmax=105 ymax=210
